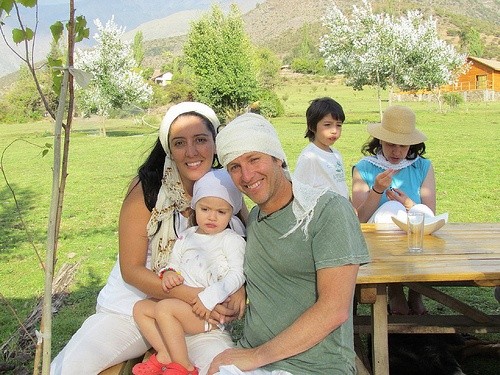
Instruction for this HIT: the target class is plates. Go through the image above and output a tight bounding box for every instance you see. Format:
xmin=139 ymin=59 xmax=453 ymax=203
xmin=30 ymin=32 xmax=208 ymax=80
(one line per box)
xmin=392 ymin=209 xmax=449 ymax=236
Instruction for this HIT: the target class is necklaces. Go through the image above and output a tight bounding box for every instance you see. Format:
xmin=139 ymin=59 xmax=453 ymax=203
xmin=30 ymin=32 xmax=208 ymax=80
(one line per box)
xmin=257 ymin=178 xmax=295 ymax=222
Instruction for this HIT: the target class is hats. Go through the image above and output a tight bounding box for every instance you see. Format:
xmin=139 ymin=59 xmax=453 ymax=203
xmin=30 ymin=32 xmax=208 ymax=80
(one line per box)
xmin=368 ymin=106 xmax=426 ymax=146
xmin=158 ymin=101 xmax=222 ymax=159
xmin=214 ymin=113 xmax=287 ymax=166
xmin=190 ymin=169 xmax=243 ymax=214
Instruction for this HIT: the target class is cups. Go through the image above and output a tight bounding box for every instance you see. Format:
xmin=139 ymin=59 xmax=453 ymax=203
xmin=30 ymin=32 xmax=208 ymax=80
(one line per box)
xmin=407 ymin=212 xmax=424 ymax=254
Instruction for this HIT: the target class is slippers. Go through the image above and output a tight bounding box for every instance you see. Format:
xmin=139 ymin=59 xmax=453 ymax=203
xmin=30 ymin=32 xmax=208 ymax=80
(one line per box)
xmin=161 ymin=363 xmax=199 ymax=375
xmin=132 ymin=353 xmax=166 ymax=375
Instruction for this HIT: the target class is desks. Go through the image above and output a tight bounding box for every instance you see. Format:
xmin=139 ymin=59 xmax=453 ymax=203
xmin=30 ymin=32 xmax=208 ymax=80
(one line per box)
xmin=356 ymin=223 xmax=500 ymax=375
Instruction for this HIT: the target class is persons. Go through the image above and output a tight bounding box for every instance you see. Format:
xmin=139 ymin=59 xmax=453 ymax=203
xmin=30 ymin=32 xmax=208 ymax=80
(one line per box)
xmin=132 ymin=169 xmax=247 ymax=375
xmin=292 ymin=96 xmax=352 ymax=202
xmin=350 ymin=105 xmax=437 ymax=317
xmin=50 ymin=102 xmax=249 ymax=375
xmin=205 ymin=112 xmax=373 ymax=375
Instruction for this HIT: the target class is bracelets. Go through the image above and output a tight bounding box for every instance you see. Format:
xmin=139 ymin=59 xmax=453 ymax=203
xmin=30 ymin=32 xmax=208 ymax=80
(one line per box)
xmin=159 ymin=267 xmax=181 ymax=279
xmin=372 ymin=186 xmax=384 ymax=195
